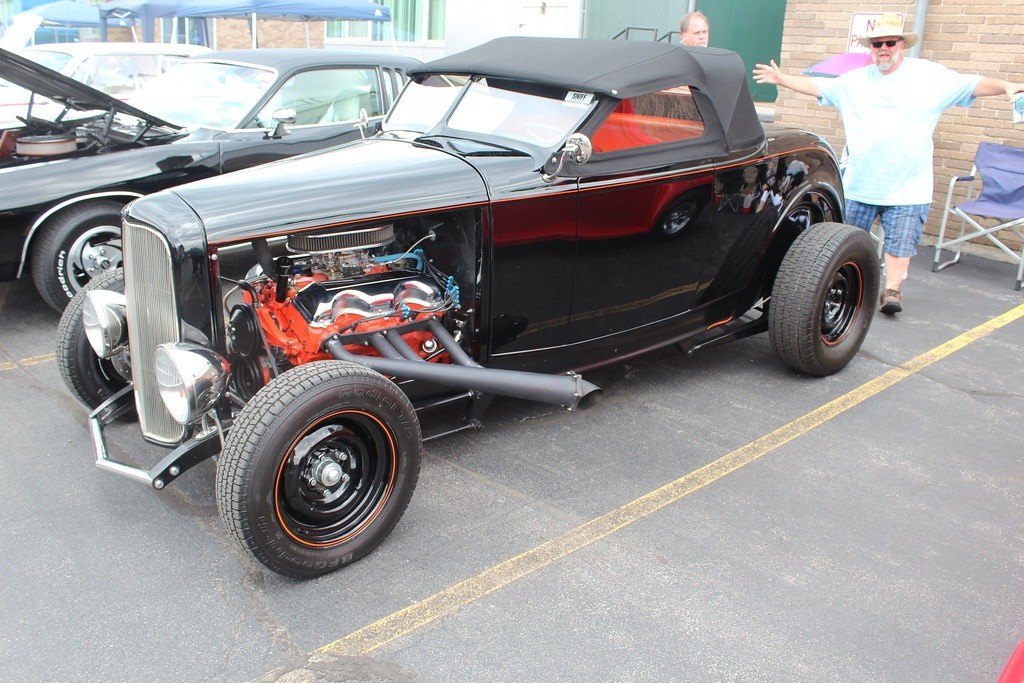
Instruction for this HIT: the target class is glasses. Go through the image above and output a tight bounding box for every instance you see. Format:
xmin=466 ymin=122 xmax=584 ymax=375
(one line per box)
xmin=872 ymin=39 xmax=901 ymax=48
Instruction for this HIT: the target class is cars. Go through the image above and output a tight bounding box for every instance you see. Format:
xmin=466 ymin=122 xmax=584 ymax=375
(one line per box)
xmin=52 ymin=32 xmax=881 ymax=581
xmin=0 ymin=35 xmax=464 ymax=315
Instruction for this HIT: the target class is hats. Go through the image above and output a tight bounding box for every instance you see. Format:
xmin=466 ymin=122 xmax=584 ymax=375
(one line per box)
xmin=856 ymin=14 xmax=919 ymax=49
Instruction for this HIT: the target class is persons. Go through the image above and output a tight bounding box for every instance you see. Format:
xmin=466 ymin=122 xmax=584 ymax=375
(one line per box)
xmin=680 ymin=12 xmax=709 ymax=47
xmin=753 ymin=12 xmax=1023 ymax=316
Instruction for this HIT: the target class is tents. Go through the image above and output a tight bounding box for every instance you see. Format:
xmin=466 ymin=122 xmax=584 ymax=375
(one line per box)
xmin=11 ymin=0 xmax=139 ymax=46
xmin=100 ymin=0 xmax=391 ymax=50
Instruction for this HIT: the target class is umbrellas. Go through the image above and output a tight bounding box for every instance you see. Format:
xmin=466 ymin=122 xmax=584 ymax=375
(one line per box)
xmin=801 ymin=53 xmax=873 ymax=78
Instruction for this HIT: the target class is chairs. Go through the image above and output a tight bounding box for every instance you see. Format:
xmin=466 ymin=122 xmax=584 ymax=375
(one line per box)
xmin=932 ymin=142 xmax=1024 ymax=290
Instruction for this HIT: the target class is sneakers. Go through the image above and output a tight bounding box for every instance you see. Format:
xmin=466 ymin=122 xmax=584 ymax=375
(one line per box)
xmin=879 ymin=288 xmax=903 ymax=314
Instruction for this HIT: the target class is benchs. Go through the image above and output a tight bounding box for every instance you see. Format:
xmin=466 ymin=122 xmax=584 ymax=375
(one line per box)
xmin=591 ymin=112 xmax=705 ymax=157
